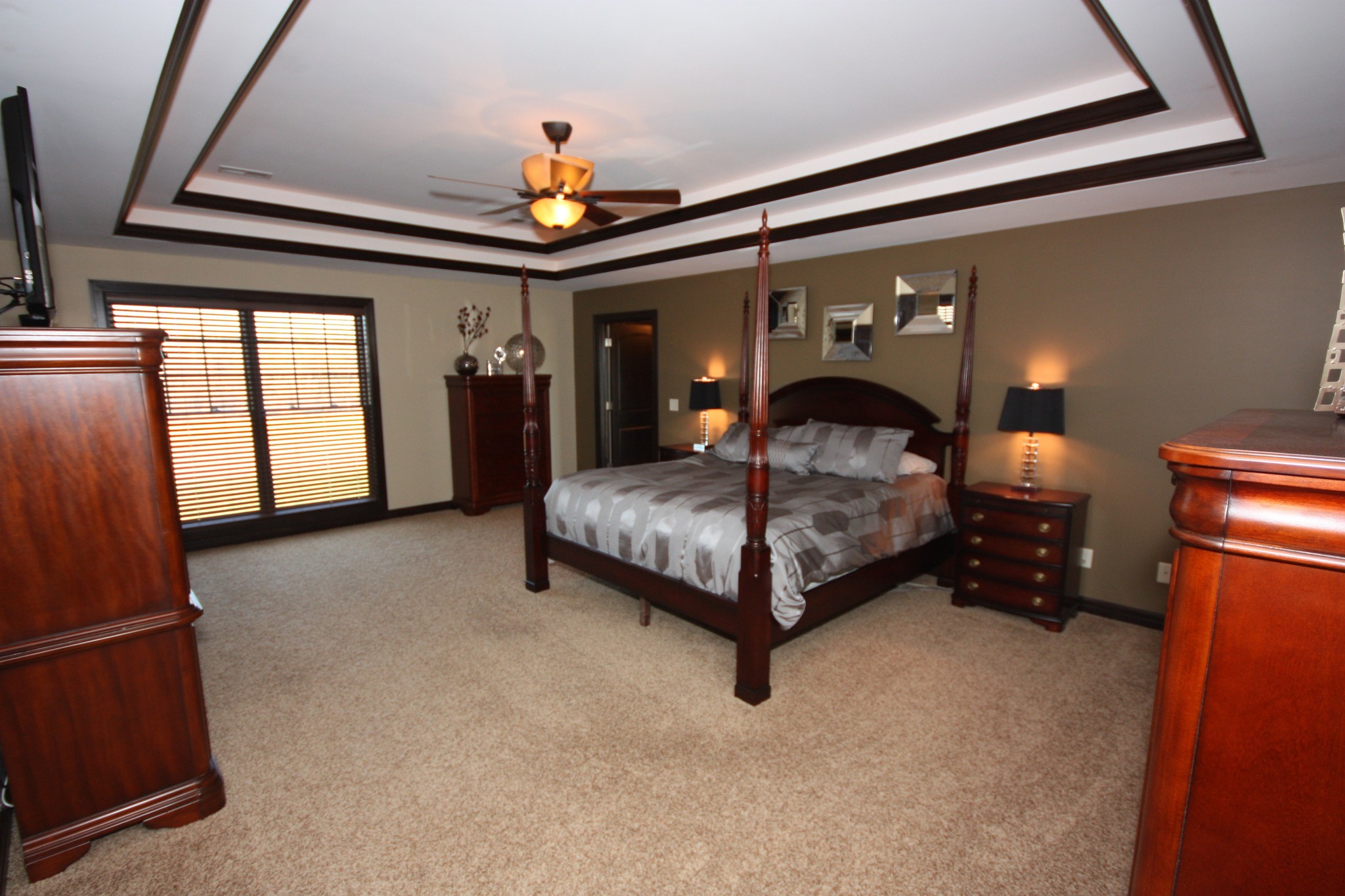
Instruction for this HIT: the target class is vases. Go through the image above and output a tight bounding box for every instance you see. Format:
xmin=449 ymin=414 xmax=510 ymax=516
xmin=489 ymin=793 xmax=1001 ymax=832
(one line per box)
xmin=454 ymin=350 xmax=479 ymax=375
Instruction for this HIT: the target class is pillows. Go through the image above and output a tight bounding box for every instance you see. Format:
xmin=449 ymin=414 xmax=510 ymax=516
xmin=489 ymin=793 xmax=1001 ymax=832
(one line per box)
xmin=895 ymin=451 xmax=937 ymax=475
xmin=802 ymin=419 xmax=914 ymax=484
xmin=765 ymin=436 xmax=817 ymax=476
xmin=709 ymin=423 xmax=794 ymax=464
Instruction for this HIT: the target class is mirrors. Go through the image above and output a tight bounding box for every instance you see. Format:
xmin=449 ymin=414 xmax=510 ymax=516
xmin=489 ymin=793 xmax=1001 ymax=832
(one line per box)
xmin=895 ymin=270 xmax=958 ymax=336
xmin=821 ymin=303 xmax=873 ymax=362
xmin=767 ymin=286 xmax=807 ymax=340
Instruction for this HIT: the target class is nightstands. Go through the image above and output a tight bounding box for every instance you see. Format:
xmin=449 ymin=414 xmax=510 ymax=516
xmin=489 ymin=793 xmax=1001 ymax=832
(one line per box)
xmin=659 ymin=443 xmax=716 ymax=461
xmin=951 ymin=482 xmax=1092 ymax=632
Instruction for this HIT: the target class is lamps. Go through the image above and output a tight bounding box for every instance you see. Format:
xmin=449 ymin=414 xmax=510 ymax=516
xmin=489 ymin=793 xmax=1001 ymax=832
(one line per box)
xmin=529 ymin=197 xmax=585 ymax=230
xmin=999 ymin=380 xmax=1066 ymax=489
xmin=689 ymin=377 xmax=721 ymax=450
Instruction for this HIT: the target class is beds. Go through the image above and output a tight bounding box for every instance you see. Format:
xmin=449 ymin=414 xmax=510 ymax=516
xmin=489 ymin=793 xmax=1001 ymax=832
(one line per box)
xmin=520 ymin=207 xmax=977 ymax=706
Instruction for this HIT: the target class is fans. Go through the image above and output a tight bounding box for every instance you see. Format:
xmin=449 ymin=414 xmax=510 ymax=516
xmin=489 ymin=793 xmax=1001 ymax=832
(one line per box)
xmin=426 ymin=120 xmax=682 ymax=229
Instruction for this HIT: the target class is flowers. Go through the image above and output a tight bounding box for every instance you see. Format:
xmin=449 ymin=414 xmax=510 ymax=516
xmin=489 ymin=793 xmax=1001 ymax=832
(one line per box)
xmin=456 ymin=304 xmax=490 ymax=349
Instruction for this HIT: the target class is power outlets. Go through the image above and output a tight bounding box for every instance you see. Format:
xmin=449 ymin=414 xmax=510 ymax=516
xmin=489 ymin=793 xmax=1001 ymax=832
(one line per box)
xmin=1080 ymin=548 xmax=1093 ymax=568
xmin=1157 ymin=562 xmax=1171 ymax=585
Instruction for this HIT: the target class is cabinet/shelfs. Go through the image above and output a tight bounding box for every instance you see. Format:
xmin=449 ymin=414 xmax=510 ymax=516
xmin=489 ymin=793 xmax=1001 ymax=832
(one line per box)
xmin=0 ymin=328 xmax=228 ymax=885
xmin=443 ymin=374 xmax=552 ymax=516
xmin=1129 ymin=406 xmax=1345 ymax=896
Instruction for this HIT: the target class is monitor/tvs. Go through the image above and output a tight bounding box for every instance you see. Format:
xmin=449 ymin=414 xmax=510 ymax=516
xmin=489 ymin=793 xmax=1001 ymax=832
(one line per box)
xmin=1 ymin=85 xmax=57 ymax=327
